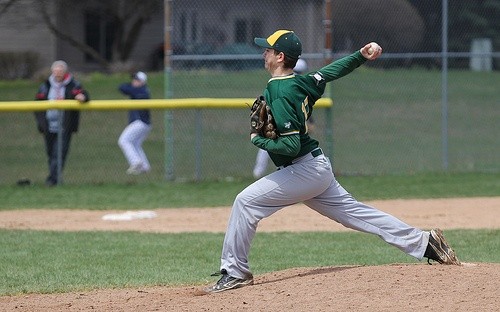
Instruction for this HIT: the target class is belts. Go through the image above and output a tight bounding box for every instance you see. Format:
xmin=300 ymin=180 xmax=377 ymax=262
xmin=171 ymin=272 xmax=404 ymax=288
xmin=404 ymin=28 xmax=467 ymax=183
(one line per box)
xmin=281 ymin=147 xmax=323 ymax=170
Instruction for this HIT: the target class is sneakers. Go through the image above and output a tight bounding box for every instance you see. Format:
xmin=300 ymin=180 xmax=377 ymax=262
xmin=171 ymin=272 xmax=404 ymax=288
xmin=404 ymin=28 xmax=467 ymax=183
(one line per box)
xmin=423 ymin=228 xmax=463 ymax=266
xmin=207 ymin=267 xmax=255 ymax=293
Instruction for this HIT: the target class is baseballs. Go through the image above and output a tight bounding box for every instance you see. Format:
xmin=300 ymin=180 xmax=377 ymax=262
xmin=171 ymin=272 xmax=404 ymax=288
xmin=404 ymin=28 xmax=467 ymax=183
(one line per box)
xmin=367 ymin=42 xmax=379 ymax=54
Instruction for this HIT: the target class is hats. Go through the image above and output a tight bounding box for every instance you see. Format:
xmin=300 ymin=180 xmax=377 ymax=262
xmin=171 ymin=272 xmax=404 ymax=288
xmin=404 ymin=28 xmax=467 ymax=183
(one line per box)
xmin=129 ymin=71 xmax=148 ymax=84
xmin=254 ymin=30 xmax=304 ymax=59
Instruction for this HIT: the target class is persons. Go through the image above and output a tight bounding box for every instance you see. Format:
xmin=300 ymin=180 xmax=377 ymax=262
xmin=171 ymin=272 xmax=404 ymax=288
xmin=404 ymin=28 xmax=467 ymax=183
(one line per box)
xmin=34 ymin=60 xmax=90 ymax=186
xmin=201 ymin=29 xmax=461 ymax=294
xmin=119 ymin=71 xmax=152 ymax=175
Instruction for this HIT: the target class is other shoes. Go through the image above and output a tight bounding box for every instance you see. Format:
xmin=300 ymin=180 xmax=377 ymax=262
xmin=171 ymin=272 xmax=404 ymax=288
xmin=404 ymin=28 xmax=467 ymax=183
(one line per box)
xmin=126 ymin=164 xmax=152 ymax=176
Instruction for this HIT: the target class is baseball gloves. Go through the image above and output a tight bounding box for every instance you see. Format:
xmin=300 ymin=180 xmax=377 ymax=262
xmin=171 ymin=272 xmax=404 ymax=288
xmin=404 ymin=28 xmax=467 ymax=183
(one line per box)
xmin=245 ymin=95 xmax=277 ymax=140
